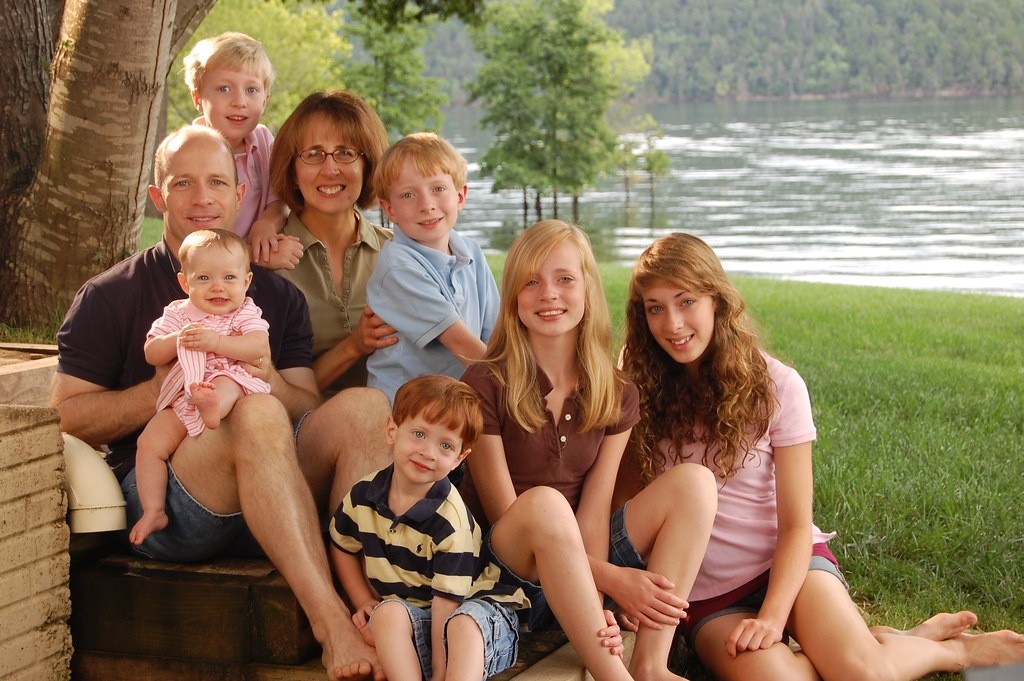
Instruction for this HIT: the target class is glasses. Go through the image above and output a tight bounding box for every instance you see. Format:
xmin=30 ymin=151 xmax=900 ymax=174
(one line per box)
xmin=295 ymin=147 xmax=363 ymax=166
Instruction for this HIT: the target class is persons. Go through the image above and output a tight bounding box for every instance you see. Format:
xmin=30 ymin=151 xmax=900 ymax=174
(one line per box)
xmin=609 ymin=230 xmax=1024 ymax=681
xmin=55 ymin=32 xmax=641 ymax=680
xmin=457 ymin=218 xmax=719 ymax=680
